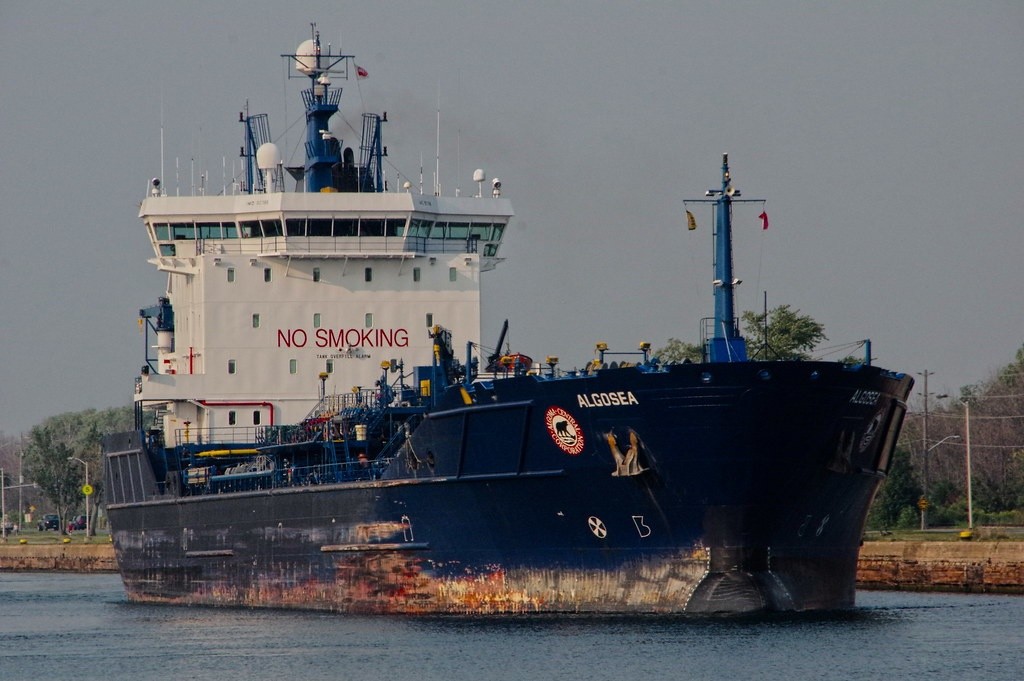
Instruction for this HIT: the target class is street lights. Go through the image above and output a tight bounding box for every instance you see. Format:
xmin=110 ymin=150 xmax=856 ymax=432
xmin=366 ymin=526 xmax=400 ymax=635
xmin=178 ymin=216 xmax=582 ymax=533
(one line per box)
xmin=921 ymin=435 xmax=960 ymax=532
xmin=68 ymin=457 xmax=89 ymax=537
xmin=936 ymin=393 xmax=973 ymax=529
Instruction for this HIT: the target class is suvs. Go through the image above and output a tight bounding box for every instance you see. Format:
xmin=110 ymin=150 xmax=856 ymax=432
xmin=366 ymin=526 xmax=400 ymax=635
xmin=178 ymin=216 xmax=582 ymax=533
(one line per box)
xmin=68 ymin=515 xmax=91 ymax=533
xmin=37 ymin=514 xmax=59 ymax=531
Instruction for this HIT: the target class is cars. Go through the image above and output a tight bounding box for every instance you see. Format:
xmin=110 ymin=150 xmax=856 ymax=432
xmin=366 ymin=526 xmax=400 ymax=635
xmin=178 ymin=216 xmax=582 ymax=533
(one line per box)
xmin=0 ymin=523 xmax=18 ymax=535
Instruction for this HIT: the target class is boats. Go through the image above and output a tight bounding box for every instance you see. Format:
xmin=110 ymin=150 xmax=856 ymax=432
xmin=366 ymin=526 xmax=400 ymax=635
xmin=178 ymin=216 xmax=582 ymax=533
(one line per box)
xmin=99 ymin=18 xmax=914 ymax=623
xmin=491 ymin=353 xmax=531 ymax=374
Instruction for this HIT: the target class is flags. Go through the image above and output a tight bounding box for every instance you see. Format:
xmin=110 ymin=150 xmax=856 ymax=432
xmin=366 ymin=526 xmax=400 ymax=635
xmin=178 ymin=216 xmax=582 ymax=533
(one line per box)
xmin=686 ymin=210 xmax=697 ymax=231
xmin=356 ymin=66 xmax=369 ymax=79
xmin=759 ymin=213 xmax=768 ymax=229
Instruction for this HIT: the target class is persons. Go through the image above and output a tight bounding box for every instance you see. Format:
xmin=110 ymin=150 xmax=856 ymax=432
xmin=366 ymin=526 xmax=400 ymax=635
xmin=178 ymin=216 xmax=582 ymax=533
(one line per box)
xmin=501 ymin=354 xmax=512 ymax=379
xmin=68 ymin=516 xmax=76 ymax=532
